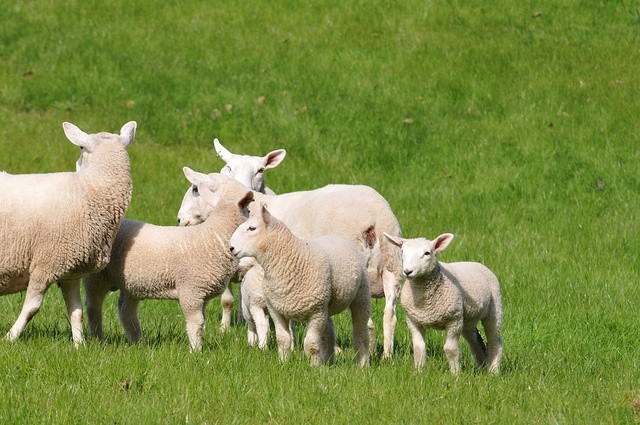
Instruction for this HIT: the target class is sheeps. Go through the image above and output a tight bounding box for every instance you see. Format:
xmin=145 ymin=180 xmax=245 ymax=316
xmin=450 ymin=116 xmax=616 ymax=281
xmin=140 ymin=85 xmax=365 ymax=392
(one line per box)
xmin=82 ymin=189 xmax=253 ymax=353
xmin=382 ymin=230 xmax=503 ymax=377
xmin=0 ymin=120 xmax=139 ymax=354
xmin=238 ymin=223 xmax=376 ymax=352
xmin=174 ymin=166 xmax=402 ymax=357
xmin=226 ymin=201 xmax=373 ymax=370
xmin=212 ymin=138 xmax=287 ymax=333
